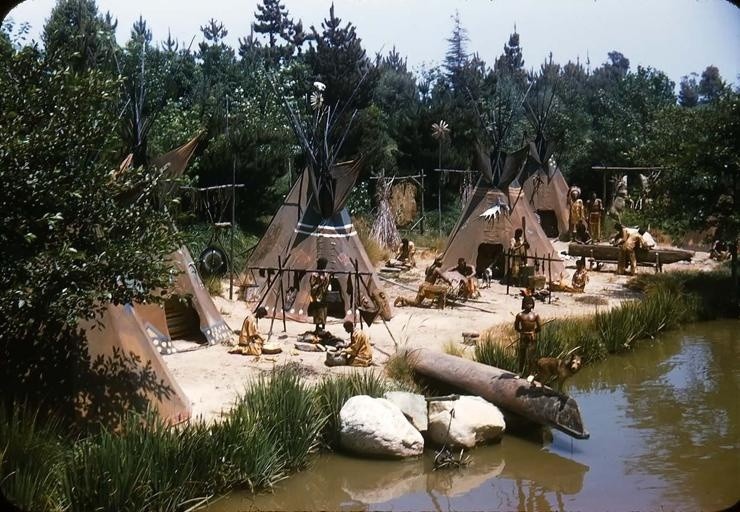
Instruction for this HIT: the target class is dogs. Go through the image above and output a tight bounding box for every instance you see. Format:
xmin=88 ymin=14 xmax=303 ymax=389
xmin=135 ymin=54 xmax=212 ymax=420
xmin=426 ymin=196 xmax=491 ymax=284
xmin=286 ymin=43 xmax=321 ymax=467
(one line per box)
xmin=526 ymin=352 xmax=585 ymax=395
xmin=481 ymin=267 xmax=492 ymax=289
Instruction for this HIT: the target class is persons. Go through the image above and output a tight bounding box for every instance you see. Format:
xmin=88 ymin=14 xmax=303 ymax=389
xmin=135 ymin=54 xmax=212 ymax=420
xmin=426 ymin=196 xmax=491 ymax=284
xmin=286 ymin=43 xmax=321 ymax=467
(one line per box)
xmin=307 ymin=257 xmax=333 ymax=336
xmin=227 ymin=307 xmax=268 ymax=356
xmin=394 ymin=228 xmax=531 ymax=310
xmin=336 ymin=321 xmax=380 ymax=367
xmin=514 ymin=296 xmax=541 ymax=376
xmin=566 ymin=186 xmax=733 ymax=293
